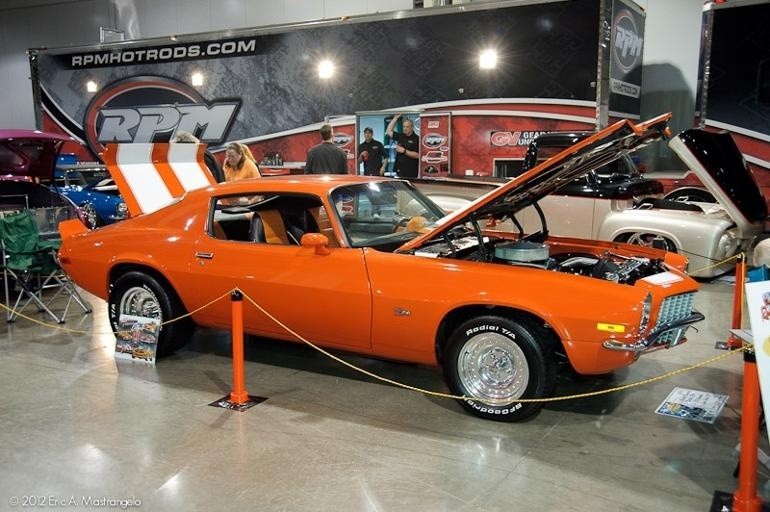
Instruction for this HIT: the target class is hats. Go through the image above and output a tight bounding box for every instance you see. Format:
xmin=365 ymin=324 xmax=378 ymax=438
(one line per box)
xmin=364 ymin=126 xmax=373 ymax=132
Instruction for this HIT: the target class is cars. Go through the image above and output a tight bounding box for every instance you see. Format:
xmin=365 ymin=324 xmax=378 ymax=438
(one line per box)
xmin=0 ymin=130 xmax=128 ymax=230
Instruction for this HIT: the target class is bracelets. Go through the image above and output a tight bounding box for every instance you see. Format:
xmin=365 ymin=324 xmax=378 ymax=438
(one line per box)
xmin=404 ymin=148 xmax=406 ymax=154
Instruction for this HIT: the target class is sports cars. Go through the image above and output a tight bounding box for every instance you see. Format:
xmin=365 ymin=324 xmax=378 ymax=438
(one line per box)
xmin=54 ymin=113 xmax=708 ymax=426
xmin=397 ymin=131 xmax=770 ymax=284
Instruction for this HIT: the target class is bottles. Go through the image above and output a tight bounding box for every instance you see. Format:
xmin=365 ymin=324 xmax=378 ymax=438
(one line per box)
xmin=275 ymin=151 xmax=279 ymax=166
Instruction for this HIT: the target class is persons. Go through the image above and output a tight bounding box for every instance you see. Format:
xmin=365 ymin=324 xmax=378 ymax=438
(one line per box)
xmin=387 ymin=113 xmax=419 ymax=177
xmin=304 ymin=124 xmax=349 ymax=173
xmin=359 ymin=126 xmax=389 ymax=176
xmin=221 ymin=142 xmax=262 ymax=180
xmin=172 ymin=130 xmax=223 ymax=182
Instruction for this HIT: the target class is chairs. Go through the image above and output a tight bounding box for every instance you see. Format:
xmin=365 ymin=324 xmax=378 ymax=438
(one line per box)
xmin=213 ymin=205 xmax=342 ymax=248
xmin=1 ymin=208 xmax=92 ymax=325
